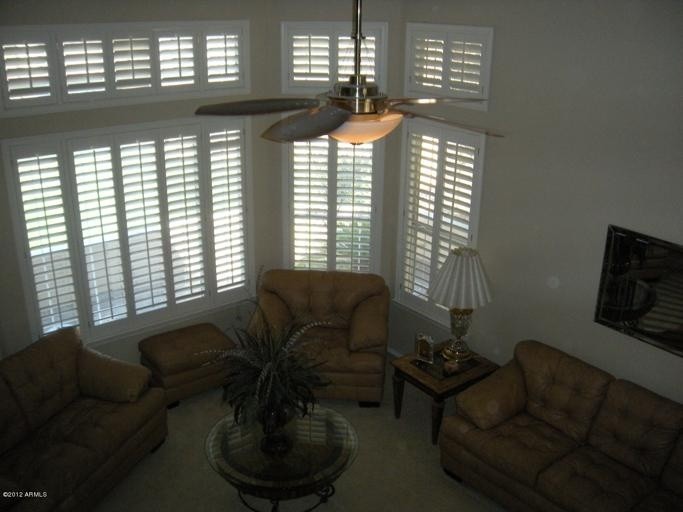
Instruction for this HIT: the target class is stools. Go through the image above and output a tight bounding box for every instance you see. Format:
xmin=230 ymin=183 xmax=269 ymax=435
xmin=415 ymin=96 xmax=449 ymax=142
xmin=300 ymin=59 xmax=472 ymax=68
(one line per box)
xmin=137 ymin=321 xmax=239 ymax=410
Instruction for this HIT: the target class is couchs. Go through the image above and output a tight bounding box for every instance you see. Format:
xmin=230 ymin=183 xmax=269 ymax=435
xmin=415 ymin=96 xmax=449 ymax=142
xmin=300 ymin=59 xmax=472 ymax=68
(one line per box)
xmin=437 ymin=338 xmax=680 ymax=511
xmin=0 ymin=321 xmax=170 ymax=512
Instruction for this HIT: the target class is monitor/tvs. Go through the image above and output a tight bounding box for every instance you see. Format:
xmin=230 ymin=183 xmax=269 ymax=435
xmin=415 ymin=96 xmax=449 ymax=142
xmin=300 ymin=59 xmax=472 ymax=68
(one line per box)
xmin=592 ymin=224 xmax=683 ymax=358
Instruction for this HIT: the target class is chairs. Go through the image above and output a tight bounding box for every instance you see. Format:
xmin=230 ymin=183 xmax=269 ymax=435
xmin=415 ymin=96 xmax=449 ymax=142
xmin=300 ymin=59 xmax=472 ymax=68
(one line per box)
xmin=247 ymin=269 xmax=390 ymax=408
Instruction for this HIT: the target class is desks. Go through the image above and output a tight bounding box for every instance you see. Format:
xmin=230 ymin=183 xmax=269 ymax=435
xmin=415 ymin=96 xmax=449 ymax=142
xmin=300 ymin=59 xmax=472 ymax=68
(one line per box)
xmin=391 ymin=340 xmax=502 ymax=445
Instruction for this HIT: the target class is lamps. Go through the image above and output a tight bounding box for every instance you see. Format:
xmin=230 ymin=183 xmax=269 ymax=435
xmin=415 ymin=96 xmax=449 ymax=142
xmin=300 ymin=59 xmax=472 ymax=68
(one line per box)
xmin=431 ymin=246 xmax=493 ymax=368
xmin=310 ymin=104 xmax=403 ymax=149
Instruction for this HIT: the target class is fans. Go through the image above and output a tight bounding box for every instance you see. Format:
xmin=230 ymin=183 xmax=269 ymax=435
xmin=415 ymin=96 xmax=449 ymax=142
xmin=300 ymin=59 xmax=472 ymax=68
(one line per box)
xmin=188 ymin=0 xmax=516 ymax=143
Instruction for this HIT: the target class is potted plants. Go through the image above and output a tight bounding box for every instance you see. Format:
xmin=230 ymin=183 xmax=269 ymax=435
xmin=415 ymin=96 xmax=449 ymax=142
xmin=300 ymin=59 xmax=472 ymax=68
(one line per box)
xmin=198 ymin=281 xmax=340 ymax=456
